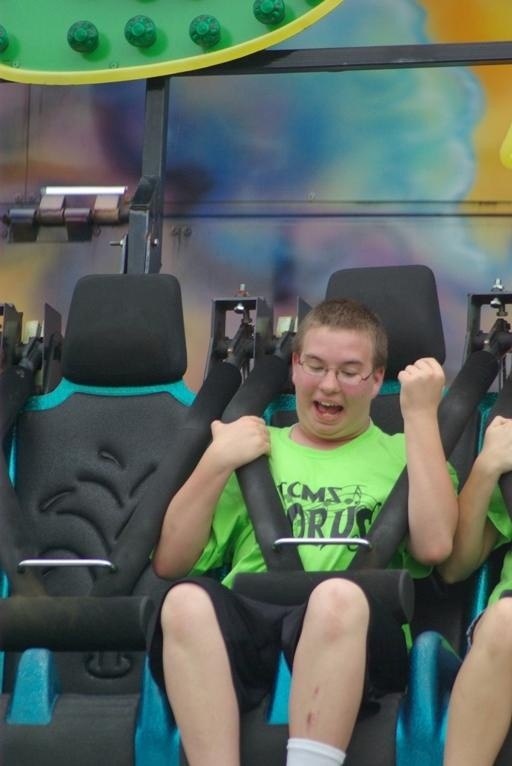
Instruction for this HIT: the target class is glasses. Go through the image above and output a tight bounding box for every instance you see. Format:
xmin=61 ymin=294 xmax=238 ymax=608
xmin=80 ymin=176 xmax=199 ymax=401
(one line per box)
xmin=297 ymin=350 xmax=374 ymax=387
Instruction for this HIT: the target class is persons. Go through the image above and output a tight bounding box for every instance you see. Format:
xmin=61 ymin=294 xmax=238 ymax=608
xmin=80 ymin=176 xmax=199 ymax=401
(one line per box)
xmin=146 ymin=298 xmax=459 ymax=766
xmin=437 ymin=415 xmax=512 ymax=766
